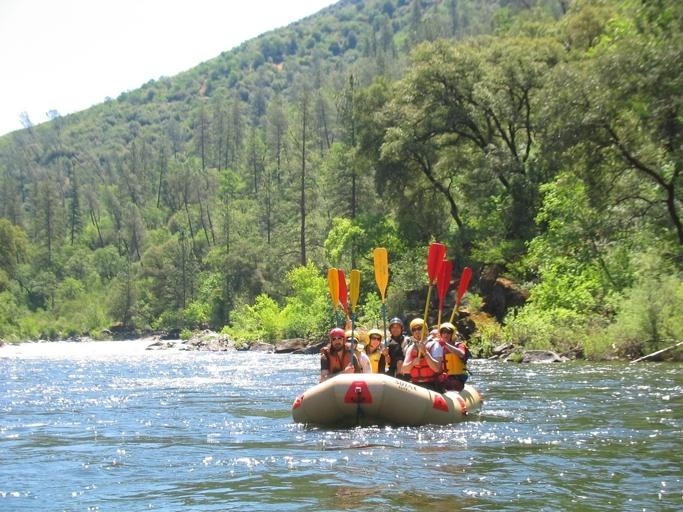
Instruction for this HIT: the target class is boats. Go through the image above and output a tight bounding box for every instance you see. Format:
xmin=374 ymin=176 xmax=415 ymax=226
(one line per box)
xmin=290 ymin=373 xmax=483 ymax=425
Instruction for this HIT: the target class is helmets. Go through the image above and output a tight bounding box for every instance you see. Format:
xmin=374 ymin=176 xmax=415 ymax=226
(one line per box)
xmin=388 ymin=317 xmax=404 ymax=330
xmin=367 ymin=328 xmax=383 ymax=340
xmin=345 ymin=330 xmax=360 ymax=341
xmin=329 ymin=328 xmax=345 ymax=338
xmin=440 ymin=322 xmax=455 ymax=333
xmin=409 ymin=318 xmax=428 ymax=335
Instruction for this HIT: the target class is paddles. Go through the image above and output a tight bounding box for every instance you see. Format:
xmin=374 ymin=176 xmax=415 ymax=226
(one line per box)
xmin=436 ymin=259 xmax=453 ymax=336
xmin=448 ymin=266 xmax=472 ymax=323
xmin=328 ymin=267 xmax=340 ymax=329
xmin=373 ymin=247 xmax=390 ymax=368
xmin=336 ymin=270 xmax=353 ymax=329
xmin=348 ymin=269 xmax=360 ymax=367
xmin=415 ymin=243 xmax=447 ymax=368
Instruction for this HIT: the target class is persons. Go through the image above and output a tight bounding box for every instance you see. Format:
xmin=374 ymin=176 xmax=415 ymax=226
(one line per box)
xmin=319 ymin=326 xmax=362 ymax=382
xmin=363 ymin=328 xmax=381 ymax=372
xmin=344 ymin=328 xmax=373 ymax=374
xmin=376 ymin=316 xmax=469 ymax=393
